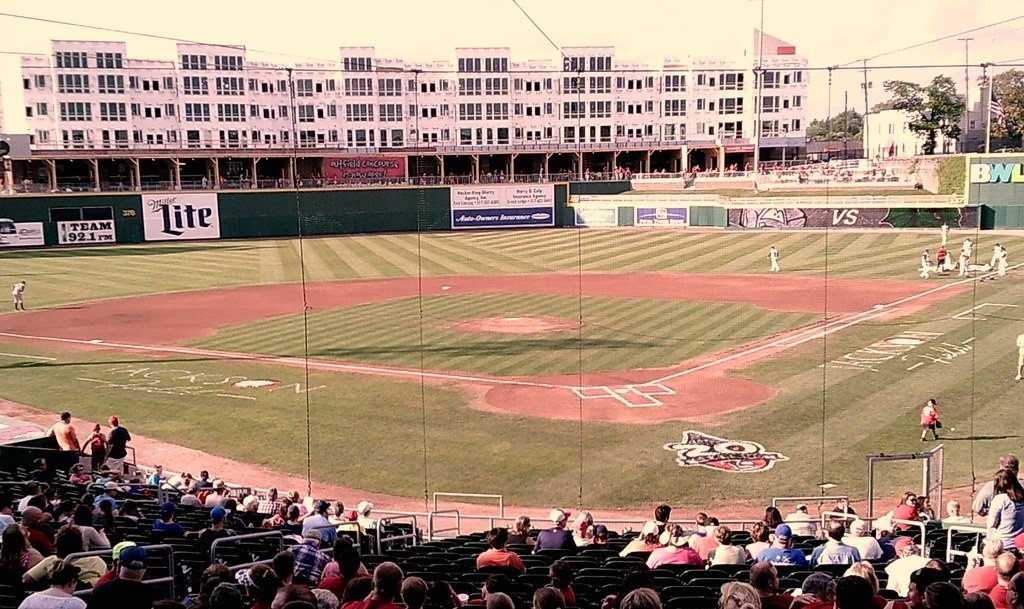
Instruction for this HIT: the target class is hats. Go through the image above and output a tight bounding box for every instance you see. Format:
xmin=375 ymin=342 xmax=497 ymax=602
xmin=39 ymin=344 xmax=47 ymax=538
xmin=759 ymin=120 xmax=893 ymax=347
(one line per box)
xmin=315 ymin=500 xmax=330 ymax=511
xmin=344 ymin=510 xmax=357 ymax=518
xmin=120 ymin=546 xmax=148 ymax=569
xmin=213 ymin=480 xmax=230 ymax=490
xmin=593 ymin=524 xmax=608 ymax=537
xmin=93 ymin=424 xmax=100 ymax=431
xmin=103 ymin=481 xmax=125 ymax=492
xmin=659 ymin=524 xmax=671 ymax=545
xmin=235 ymin=564 xmax=279 ymax=600
xmin=162 ymin=503 xmax=178 ymax=512
xmin=53 ymin=561 xmax=81 ymax=581
xmin=210 ymin=507 xmax=231 ymax=518
xmin=201 ymin=471 xmax=208 ymax=479
xmin=549 ymin=509 xmax=571 ymax=522
xmin=775 ymin=524 xmax=791 ymax=540
xmin=112 ymin=541 xmax=137 ymax=560
xmin=358 ymin=501 xmax=373 ymax=513
xmin=931 ymin=399 xmax=937 ymax=405
xmin=109 ymin=416 xmax=118 ymax=425
xmin=33 ymin=458 xmax=46 ymax=469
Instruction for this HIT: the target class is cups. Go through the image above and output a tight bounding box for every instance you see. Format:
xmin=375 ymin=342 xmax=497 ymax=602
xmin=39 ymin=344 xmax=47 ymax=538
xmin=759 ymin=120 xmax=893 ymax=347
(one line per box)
xmin=457 ymin=594 xmax=469 ymax=606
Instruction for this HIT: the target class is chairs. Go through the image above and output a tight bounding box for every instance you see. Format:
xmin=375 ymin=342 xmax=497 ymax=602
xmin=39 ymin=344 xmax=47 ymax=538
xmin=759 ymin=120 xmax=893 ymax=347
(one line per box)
xmin=0 ymin=462 xmax=1024 ymax=609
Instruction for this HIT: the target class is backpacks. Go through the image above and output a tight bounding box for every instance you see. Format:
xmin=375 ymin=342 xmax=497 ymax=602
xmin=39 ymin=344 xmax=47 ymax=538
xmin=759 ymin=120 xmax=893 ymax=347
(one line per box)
xmin=91 ymin=434 xmax=103 ymax=449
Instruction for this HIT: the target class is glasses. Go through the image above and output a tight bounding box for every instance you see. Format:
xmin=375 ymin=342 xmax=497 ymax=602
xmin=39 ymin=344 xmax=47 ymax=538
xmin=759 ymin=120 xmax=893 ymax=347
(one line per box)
xmin=908 ymin=498 xmax=916 ymax=502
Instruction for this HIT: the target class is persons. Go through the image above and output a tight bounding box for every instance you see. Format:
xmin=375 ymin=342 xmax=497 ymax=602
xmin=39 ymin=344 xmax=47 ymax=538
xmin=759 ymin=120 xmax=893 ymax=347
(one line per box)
xmin=919 ymin=399 xmax=943 ymax=442
xmin=11 ymin=280 xmax=26 ymax=311
xmin=918 ymin=223 xmax=1009 ymax=279
xmin=767 ymin=244 xmax=781 ymax=272
xmin=0 ymin=178 xmax=4 ymax=190
xmin=1014 ymin=334 xmax=1024 ymax=381
xmin=116 ymin=173 xmax=126 ymax=192
xmin=0 ymin=413 xmax=1024 ymax=609
xmin=202 ymin=155 xmax=918 ymax=190
